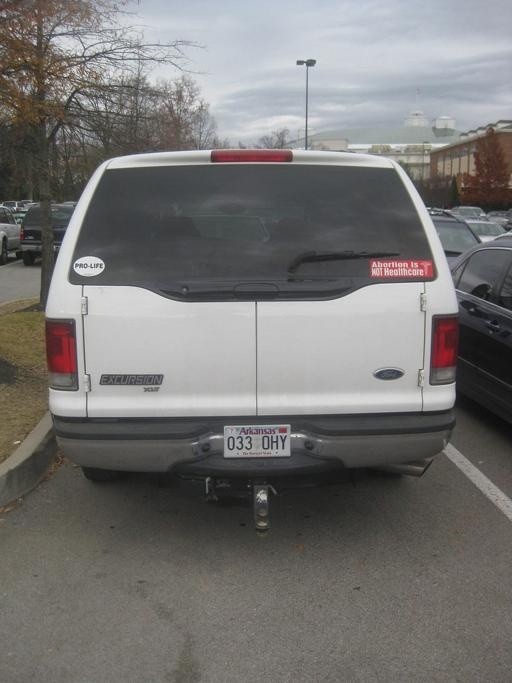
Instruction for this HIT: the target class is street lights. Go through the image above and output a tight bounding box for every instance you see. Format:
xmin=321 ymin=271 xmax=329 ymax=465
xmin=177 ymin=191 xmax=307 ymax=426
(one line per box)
xmin=295 ymin=58 xmax=317 ymax=149
